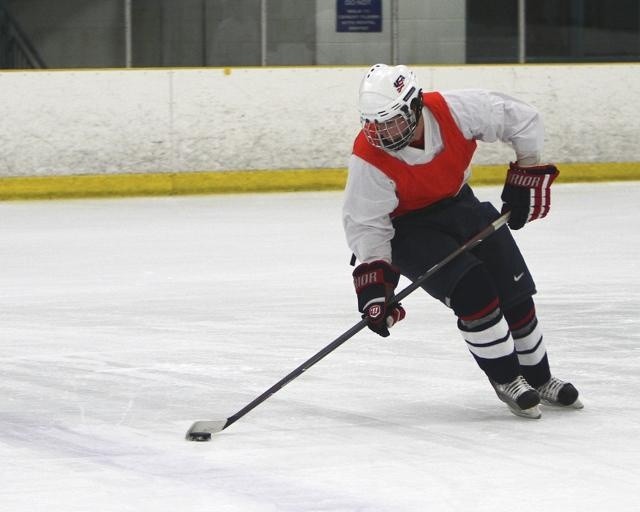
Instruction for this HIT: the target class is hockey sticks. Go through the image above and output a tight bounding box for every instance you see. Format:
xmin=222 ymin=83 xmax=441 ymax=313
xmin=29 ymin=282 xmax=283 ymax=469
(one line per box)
xmin=190 ymin=212 xmax=509 ymax=433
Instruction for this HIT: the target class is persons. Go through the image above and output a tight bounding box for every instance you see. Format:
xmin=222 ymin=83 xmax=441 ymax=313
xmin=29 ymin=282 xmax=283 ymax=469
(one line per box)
xmin=341 ymin=63 xmax=579 ymax=411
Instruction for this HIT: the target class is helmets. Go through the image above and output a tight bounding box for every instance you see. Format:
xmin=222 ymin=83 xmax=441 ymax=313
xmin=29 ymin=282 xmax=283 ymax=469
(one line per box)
xmin=357 ymin=65 xmax=423 ymax=153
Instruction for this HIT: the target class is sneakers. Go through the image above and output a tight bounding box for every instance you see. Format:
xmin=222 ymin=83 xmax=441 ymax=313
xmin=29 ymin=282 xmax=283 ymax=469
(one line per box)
xmin=487 ymin=375 xmax=540 ymax=411
xmin=534 ymin=377 xmax=579 ymax=406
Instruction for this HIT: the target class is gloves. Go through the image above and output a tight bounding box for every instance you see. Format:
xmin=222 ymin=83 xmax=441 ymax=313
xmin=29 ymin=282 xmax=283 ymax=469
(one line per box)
xmin=353 ymin=260 xmax=405 ymax=338
xmin=501 ymin=161 xmax=559 ymax=229
